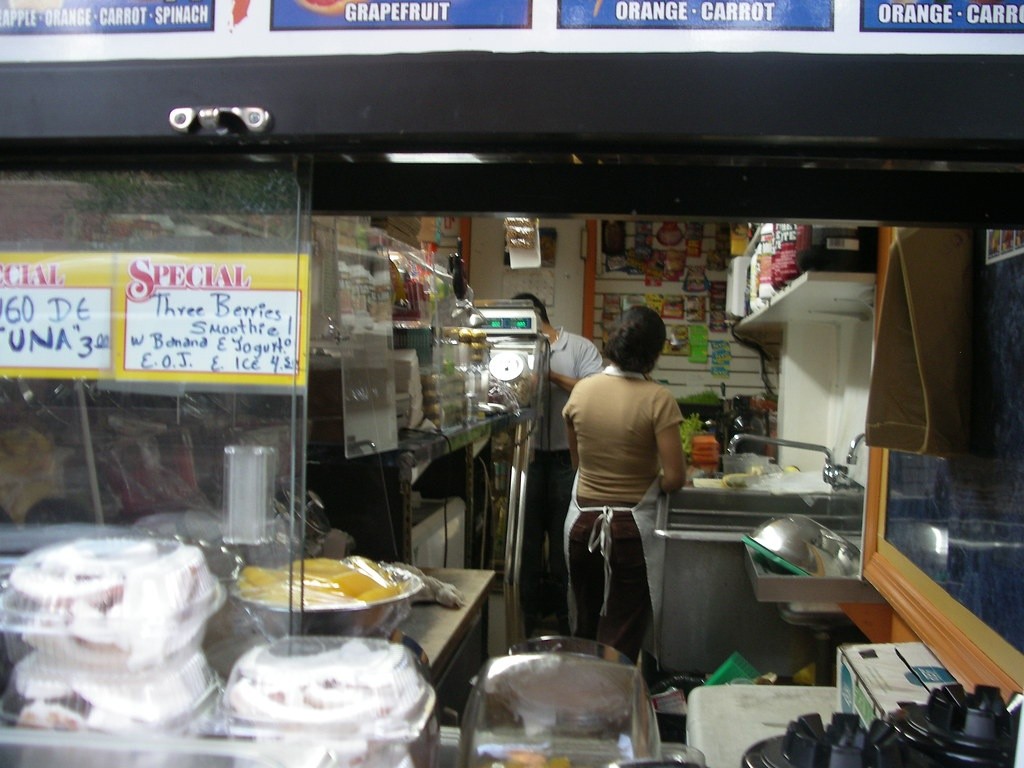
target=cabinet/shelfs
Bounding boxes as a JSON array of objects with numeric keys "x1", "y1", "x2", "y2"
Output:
[{"x1": 0, "y1": 209, "x2": 543, "y2": 597}]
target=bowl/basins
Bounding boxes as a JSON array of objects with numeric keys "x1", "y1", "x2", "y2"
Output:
[
  {"x1": 741, "y1": 514, "x2": 861, "y2": 576},
  {"x1": 509, "y1": 635, "x2": 638, "y2": 671},
  {"x1": 228, "y1": 563, "x2": 423, "y2": 644}
]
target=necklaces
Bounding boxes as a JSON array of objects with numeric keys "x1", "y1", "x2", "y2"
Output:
[{"x1": 555, "y1": 330, "x2": 559, "y2": 340}]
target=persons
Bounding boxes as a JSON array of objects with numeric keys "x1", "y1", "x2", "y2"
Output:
[
  {"x1": 561, "y1": 303, "x2": 685, "y2": 659},
  {"x1": 512, "y1": 293, "x2": 604, "y2": 634}
]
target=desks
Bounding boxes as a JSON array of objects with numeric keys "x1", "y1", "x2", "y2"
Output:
[{"x1": 388, "y1": 568, "x2": 496, "y2": 728}]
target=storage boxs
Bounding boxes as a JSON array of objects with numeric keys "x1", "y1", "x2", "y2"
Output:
[
  {"x1": 0, "y1": 538, "x2": 437, "y2": 768},
  {"x1": 838, "y1": 642, "x2": 960, "y2": 733}
]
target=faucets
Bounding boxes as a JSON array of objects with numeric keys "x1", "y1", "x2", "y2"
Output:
[
  {"x1": 726, "y1": 433, "x2": 836, "y2": 487},
  {"x1": 845, "y1": 432, "x2": 864, "y2": 465}
]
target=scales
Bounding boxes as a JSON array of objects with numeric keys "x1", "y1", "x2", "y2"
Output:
[{"x1": 472, "y1": 299, "x2": 543, "y2": 337}]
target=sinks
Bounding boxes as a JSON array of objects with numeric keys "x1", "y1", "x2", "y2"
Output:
[
  {"x1": 669, "y1": 488, "x2": 840, "y2": 516},
  {"x1": 666, "y1": 511, "x2": 854, "y2": 531}
]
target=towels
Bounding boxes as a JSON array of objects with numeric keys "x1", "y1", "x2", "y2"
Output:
[{"x1": 865, "y1": 228, "x2": 987, "y2": 459}]
